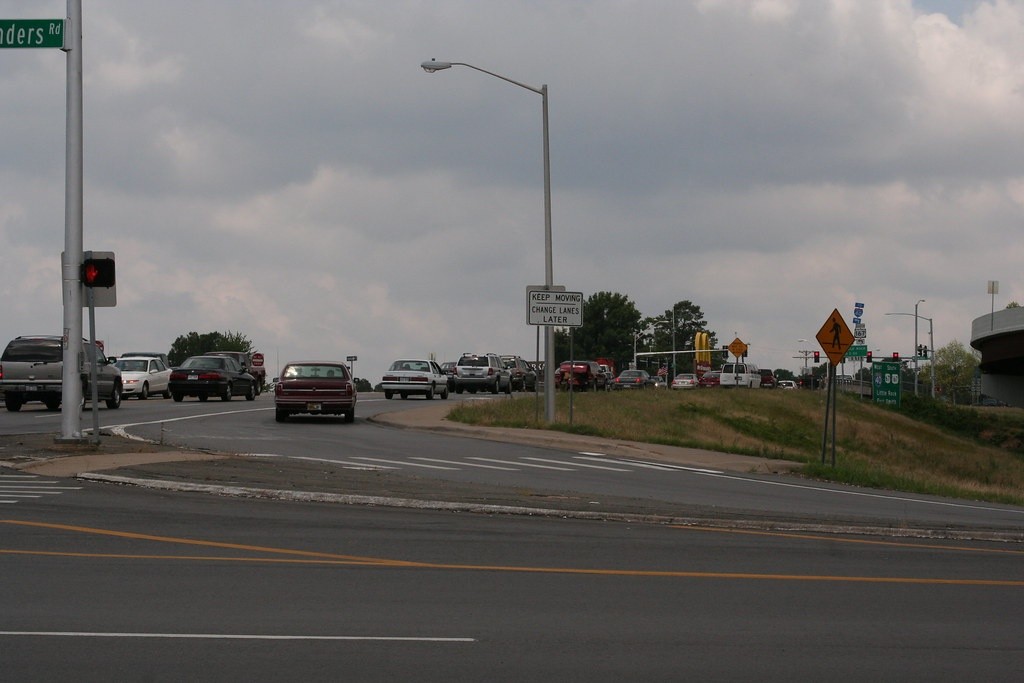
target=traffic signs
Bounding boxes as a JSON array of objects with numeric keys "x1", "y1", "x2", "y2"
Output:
[{"x1": 346, "y1": 356, "x2": 357, "y2": 362}]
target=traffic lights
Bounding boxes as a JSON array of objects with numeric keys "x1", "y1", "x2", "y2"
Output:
[
  {"x1": 893, "y1": 352, "x2": 899, "y2": 362},
  {"x1": 922, "y1": 345, "x2": 927, "y2": 358},
  {"x1": 814, "y1": 351, "x2": 819, "y2": 363},
  {"x1": 82, "y1": 259, "x2": 116, "y2": 288},
  {"x1": 866, "y1": 351, "x2": 873, "y2": 363},
  {"x1": 918, "y1": 344, "x2": 922, "y2": 357}
]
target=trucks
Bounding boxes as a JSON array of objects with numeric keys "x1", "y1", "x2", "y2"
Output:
[{"x1": 555, "y1": 356, "x2": 617, "y2": 393}]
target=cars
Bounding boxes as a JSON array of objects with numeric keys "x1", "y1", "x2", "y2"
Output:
[
  {"x1": 615, "y1": 370, "x2": 651, "y2": 391},
  {"x1": 110, "y1": 356, "x2": 175, "y2": 400},
  {"x1": 272, "y1": 360, "x2": 360, "y2": 424},
  {"x1": 439, "y1": 362, "x2": 458, "y2": 393},
  {"x1": 699, "y1": 371, "x2": 720, "y2": 388},
  {"x1": 381, "y1": 359, "x2": 449, "y2": 400},
  {"x1": 167, "y1": 355, "x2": 258, "y2": 402},
  {"x1": 672, "y1": 373, "x2": 699, "y2": 390},
  {"x1": 650, "y1": 376, "x2": 668, "y2": 391},
  {"x1": 778, "y1": 381, "x2": 799, "y2": 391}
]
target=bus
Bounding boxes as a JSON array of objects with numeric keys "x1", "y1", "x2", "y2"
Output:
[{"x1": 836, "y1": 374, "x2": 852, "y2": 386}]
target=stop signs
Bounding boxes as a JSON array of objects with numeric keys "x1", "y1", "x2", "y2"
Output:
[{"x1": 251, "y1": 353, "x2": 264, "y2": 367}]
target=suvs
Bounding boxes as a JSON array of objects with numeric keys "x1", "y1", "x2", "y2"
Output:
[
  {"x1": 453, "y1": 351, "x2": 512, "y2": 394},
  {"x1": 499, "y1": 354, "x2": 538, "y2": 393},
  {"x1": 0, "y1": 334, "x2": 124, "y2": 412},
  {"x1": 798, "y1": 374, "x2": 825, "y2": 389}
]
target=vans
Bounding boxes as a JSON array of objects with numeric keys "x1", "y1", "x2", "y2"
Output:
[
  {"x1": 758, "y1": 368, "x2": 779, "y2": 387},
  {"x1": 119, "y1": 352, "x2": 170, "y2": 368},
  {"x1": 720, "y1": 362, "x2": 761, "y2": 389},
  {"x1": 203, "y1": 351, "x2": 250, "y2": 373}
]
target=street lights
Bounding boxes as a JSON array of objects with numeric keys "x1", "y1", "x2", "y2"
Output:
[
  {"x1": 914, "y1": 299, "x2": 926, "y2": 398},
  {"x1": 420, "y1": 59, "x2": 556, "y2": 430},
  {"x1": 884, "y1": 312, "x2": 935, "y2": 399},
  {"x1": 633, "y1": 321, "x2": 668, "y2": 370}
]
